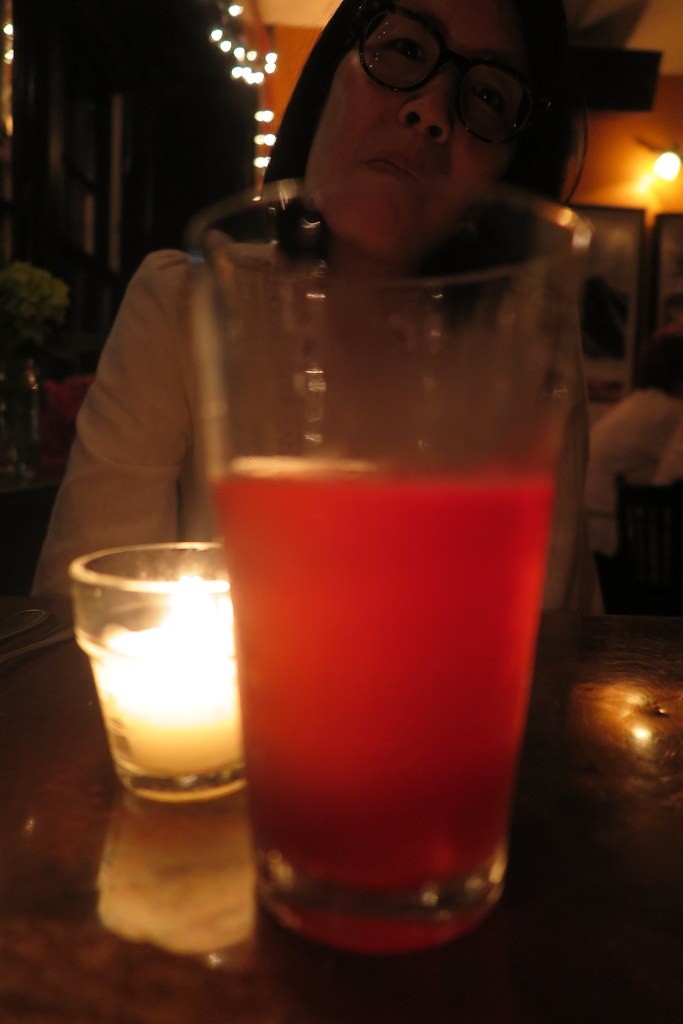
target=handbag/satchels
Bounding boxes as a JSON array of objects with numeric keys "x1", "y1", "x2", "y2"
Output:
[{"x1": 38, "y1": 374, "x2": 96, "y2": 474}]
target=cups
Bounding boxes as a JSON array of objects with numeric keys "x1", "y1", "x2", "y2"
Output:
[{"x1": 202, "y1": 186, "x2": 575, "y2": 943}]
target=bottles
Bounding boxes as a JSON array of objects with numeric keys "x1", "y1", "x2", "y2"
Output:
[{"x1": 11, "y1": 359, "x2": 41, "y2": 445}]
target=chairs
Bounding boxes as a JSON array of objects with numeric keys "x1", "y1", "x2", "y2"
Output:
[{"x1": 614, "y1": 472, "x2": 682, "y2": 619}]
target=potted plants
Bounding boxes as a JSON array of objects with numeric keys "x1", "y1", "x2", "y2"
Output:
[{"x1": 1, "y1": 260, "x2": 69, "y2": 466}]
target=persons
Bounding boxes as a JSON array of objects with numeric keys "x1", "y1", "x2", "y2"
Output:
[
  {"x1": 576, "y1": 325, "x2": 683, "y2": 603},
  {"x1": 29, "y1": 0, "x2": 606, "y2": 617}
]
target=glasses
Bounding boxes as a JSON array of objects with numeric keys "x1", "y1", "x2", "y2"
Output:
[{"x1": 342, "y1": 0, "x2": 553, "y2": 145}]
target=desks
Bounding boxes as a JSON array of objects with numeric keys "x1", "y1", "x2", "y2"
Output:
[{"x1": 0, "y1": 591, "x2": 682, "y2": 1024}]
